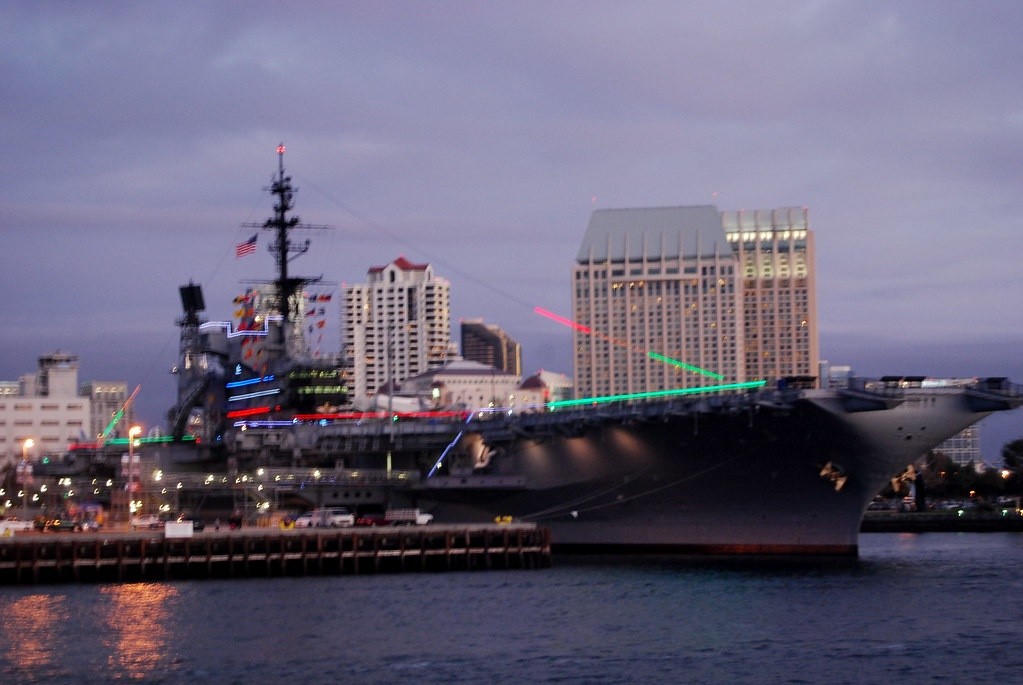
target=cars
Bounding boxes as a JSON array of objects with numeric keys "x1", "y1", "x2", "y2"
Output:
[
  {"x1": 128, "y1": 513, "x2": 161, "y2": 529},
  {"x1": 358, "y1": 513, "x2": 387, "y2": 529}
]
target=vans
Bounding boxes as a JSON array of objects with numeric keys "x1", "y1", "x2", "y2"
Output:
[{"x1": 311, "y1": 505, "x2": 357, "y2": 528}]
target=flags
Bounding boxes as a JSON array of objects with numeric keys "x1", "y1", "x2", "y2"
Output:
[
  {"x1": 235, "y1": 231, "x2": 259, "y2": 256},
  {"x1": 232, "y1": 288, "x2": 333, "y2": 378}
]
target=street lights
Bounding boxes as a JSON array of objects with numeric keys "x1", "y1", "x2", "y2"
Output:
[
  {"x1": 129, "y1": 425, "x2": 143, "y2": 515},
  {"x1": 22, "y1": 438, "x2": 35, "y2": 520}
]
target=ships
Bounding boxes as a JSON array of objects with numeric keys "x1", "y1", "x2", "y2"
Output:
[{"x1": 22, "y1": 131, "x2": 1023, "y2": 566}]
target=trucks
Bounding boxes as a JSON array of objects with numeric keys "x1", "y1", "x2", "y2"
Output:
[{"x1": 383, "y1": 507, "x2": 432, "y2": 526}]
